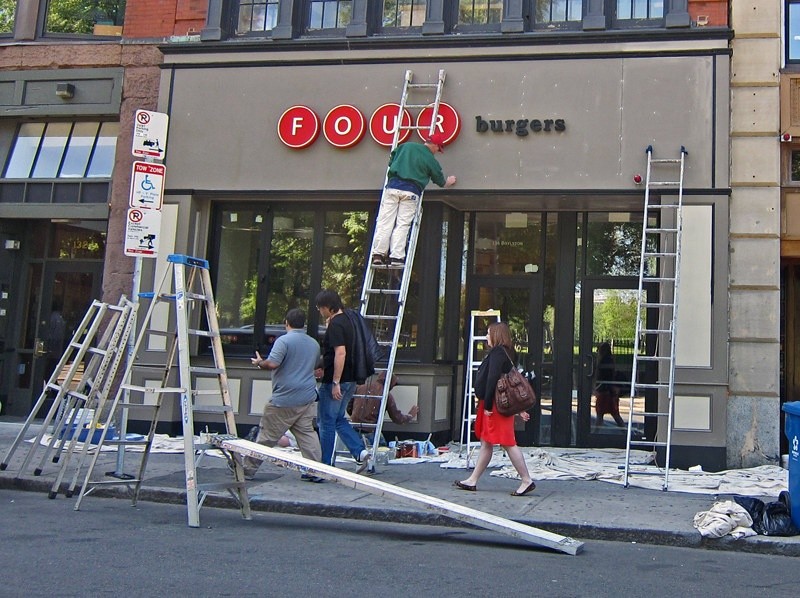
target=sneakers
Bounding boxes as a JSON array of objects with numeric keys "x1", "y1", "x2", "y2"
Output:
[{"x1": 355, "y1": 450, "x2": 370, "y2": 474}]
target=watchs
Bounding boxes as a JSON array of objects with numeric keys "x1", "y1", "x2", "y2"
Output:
[{"x1": 332, "y1": 382, "x2": 338, "y2": 386}]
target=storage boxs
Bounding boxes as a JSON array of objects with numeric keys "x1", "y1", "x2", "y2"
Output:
[{"x1": 60, "y1": 422, "x2": 116, "y2": 444}]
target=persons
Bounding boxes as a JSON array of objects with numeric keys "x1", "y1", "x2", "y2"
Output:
[
  {"x1": 227, "y1": 308, "x2": 321, "y2": 481},
  {"x1": 454, "y1": 322, "x2": 536, "y2": 496},
  {"x1": 347, "y1": 371, "x2": 420, "y2": 447},
  {"x1": 315, "y1": 288, "x2": 370, "y2": 474},
  {"x1": 596, "y1": 341, "x2": 626, "y2": 427},
  {"x1": 373, "y1": 134, "x2": 456, "y2": 265},
  {"x1": 43, "y1": 300, "x2": 64, "y2": 397}
]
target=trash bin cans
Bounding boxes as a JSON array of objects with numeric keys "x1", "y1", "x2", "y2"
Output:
[{"x1": 782, "y1": 402, "x2": 800, "y2": 531}]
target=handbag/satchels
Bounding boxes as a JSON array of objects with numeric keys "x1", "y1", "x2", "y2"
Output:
[{"x1": 493, "y1": 346, "x2": 538, "y2": 417}]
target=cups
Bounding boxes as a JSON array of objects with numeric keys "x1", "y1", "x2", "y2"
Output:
[
  {"x1": 782, "y1": 454, "x2": 789, "y2": 469},
  {"x1": 689, "y1": 465, "x2": 702, "y2": 471}
]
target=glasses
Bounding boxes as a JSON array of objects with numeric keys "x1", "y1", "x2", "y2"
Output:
[{"x1": 315, "y1": 304, "x2": 320, "y2": 311}]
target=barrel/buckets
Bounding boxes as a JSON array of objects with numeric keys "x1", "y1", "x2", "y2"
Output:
[
  {"x1": 65, "y1": 407, "x2": 95, "y2": 425},
  {"x1": 376, "y1": 440, "x2": 429, "y2": 467}
]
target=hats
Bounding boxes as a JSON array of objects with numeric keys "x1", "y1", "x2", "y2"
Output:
[{"x1": 426, "y1": 134, "x2": 444, "y2": 154}]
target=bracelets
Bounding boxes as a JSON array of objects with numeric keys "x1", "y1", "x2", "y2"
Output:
[{"x1": 258, "y1": 364, "x2": 261, "y2": 369}]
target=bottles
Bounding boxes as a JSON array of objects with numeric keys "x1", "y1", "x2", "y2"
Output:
[{"x1": 438, "y1": 447, "x2": 449, "y2": 455}]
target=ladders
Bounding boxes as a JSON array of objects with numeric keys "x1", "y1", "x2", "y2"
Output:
[
  {"x1": 1, "y1": 292, "x2": 139, "y2": 500},
  {"x1": 330, "y1": 69, "x2": 447, "y2": 472},
  {"x1": 74, "y1": 255, "x2": 252, "y2": 527},
  {"x1": 623, "y1": 145, "x2": 687, "y2": 491},
  {"x1": 459, "y1": 310, "x2": 507, "y2": 470}
]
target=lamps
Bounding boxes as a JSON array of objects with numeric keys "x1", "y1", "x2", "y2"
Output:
[{"x1": 56, "y1": 83, "x2": 74, "y2": 98}]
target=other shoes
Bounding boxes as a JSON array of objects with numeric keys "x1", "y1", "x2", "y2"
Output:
[
  {"x1": 227, "y1": 462, "x2": 256, "y2": 480},
  {"x1": 390, "y1": 258, "x2": 404, "y2": 266},
  {"x1": 372, "y1": 254, "x2": 386, "y2": 265},
  {"x1": 453, "y1": 479, "x2": 477, "y2": 492},
  {"x1": 300, "y1": 473, "x2": 325, "y2": 483},
  {"x1": 509, "y1": 481, "x2": 537, "y2": 496}
]
